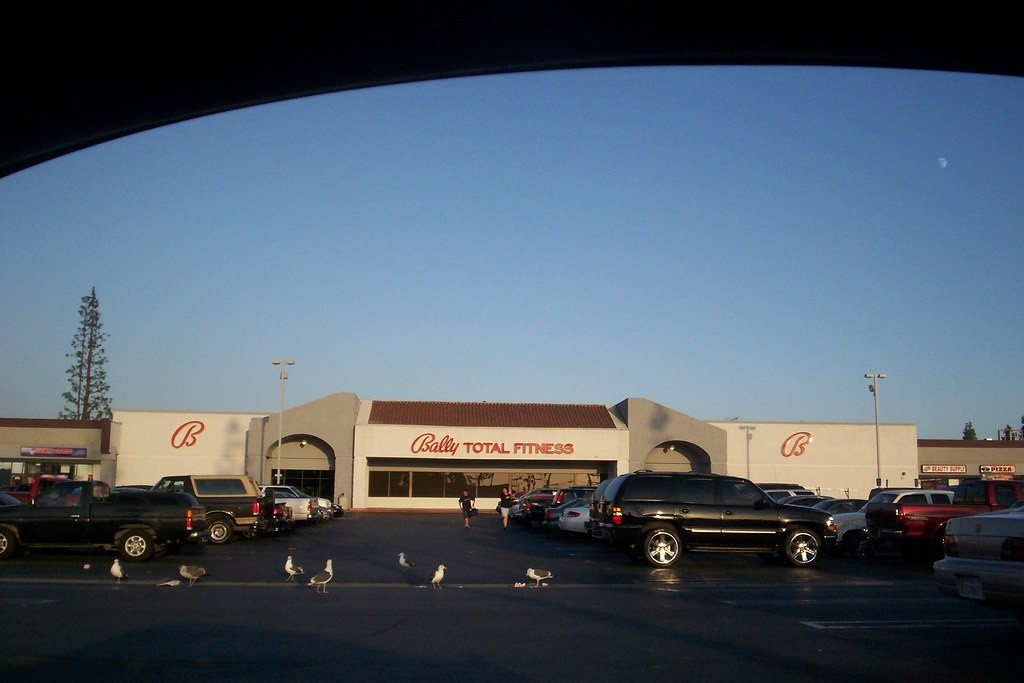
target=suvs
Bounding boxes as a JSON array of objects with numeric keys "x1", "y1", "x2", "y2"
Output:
[
  {"x1": 148, "y1": 474, "x2": 265, "y2": 544},
  {"x1": 597, "y1": 468, "x2": 836, "y2": 569}
]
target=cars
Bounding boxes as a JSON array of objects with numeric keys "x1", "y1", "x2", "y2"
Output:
[
  {"x1": 498, "y1": 487, "x2": 593, "y2": 544},
  {"x1": 933, "y1": 500, "x2": 1024, "y2": 625},
  {"x1": 757, "y1": 483, "x2": 957, "y2": 558},
  {"x1": 259, "y1": 484, "x2": 344, "y2": 534}
]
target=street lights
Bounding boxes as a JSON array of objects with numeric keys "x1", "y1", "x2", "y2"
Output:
[
  {"x1": 864, "y1": 374, "x2": 886, "y2": 489},
  {"x1": 273, "y1": 361, "x2": 295, "y2": 483}
]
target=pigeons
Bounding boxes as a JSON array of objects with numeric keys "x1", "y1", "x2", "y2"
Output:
[
  {"x1": 525, "y1": 568, "x2": 555, "y2": 587},
  {"x1": 304, "y1": 558, "x2": 334, "y2": 593},
  {"x1": 397, "y1": 552, "x2": 416, "y2": 569},
  {"x1": 110, "y1": 559, "x2": 128, "y2": 584},
  {"x1": 428, "y1": 564, "x2": 448, "y2": 589},
  {"x1": 284, "y1": 555, "x2": 304, "y2": 582},
  {"x1": 178, "y1": 564, "x2": 211, "y2": 587}
]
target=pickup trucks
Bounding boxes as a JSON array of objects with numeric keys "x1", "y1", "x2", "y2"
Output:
[
  {"x1": 0, "y1": 480, "x2": 206, "y2": 563},
  {"x1": 867, "y1": 480, "x2": 1024, "y2": 562},
  {"x1": 4, "y1": 475, "x2": 81, "y2": 506}
]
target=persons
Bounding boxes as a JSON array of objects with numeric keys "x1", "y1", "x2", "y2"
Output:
[
  {"x1": 458, "y1": 490, "x2": 475, "y2": 527},
  {"x1": 500, "y1": 487, "x2": 515, "y2": 528}
]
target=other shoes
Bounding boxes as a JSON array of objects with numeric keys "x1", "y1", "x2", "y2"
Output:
[{"x1": 465, "y1": 525, "x2": 469, "y2": 528}]
